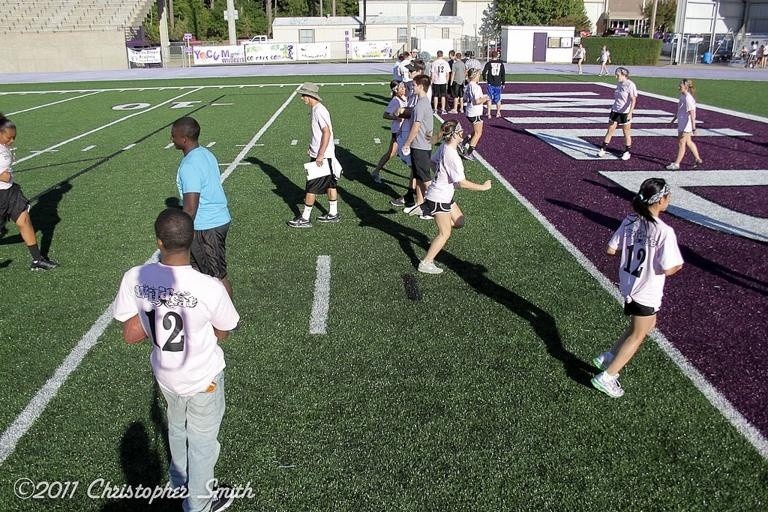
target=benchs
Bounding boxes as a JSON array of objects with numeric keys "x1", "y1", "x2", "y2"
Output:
[{"x1": 0, "y1": 0, "x2": 157, "y2": 42}]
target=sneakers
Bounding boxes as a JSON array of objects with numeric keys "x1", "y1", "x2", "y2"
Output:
[
  {"x1": 463, "y1": 152, "x2": 475, "y2": 160},
  {"x1": 370, "y1": 172, "x2": 385, "y2": 183},
  {"x1": 666, "y1": 163, "x2": 679, "y2": 170},
  {"x1": 597, "y1": 148, "x2": 606, "y2": 157},
  {"x1": 457, "y1": 142, "x2": 465, "y2": 153},
  {"x1": 288, "y1": 215, "x2": 313, "y2": 228},
  {"x1": 432, "y1": 108, "x2": 464, "y2": 117},
  {"x1": 315, "y1": 212, "x2": 339, "y2": 223},
  {"x1": 418, "y1": 260, "x2": 443, "y2": 274},
  {"x1": 485, "y1": 112, "x2": 501, "y2": 119},
  {"x1": 689, "y1": 159, "x2": 702, "y2": 168},
  {"x1": 391, "y1": 196, "x2": 435, "y2": 219},
  {"x1": 30, "y1": 258, "x2": 59, "y2": 272},
  {"x1": 590, "y1": 351, "x2": 624, "y2": 397},
  {"x1": 211, "y1": 483, "x2": 235, "y2": 512},
  {"x1": 622, "y1": 152, "x2": 631, "y2": 160}
]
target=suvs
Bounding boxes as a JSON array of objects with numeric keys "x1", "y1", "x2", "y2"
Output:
[{"x1": 606, "y1": 28, "x2": 631, "y2": 37}]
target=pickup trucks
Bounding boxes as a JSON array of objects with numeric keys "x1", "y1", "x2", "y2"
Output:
[{"x1": 241, "y1": 34, "x2": 273, "y2": 45}]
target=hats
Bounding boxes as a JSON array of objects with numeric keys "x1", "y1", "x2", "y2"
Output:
[
  {"x1": 410, "y1": 49, "x2": 419, "y2": 53},
  {"x1": 468, "y1": 68, "x2": 480, "y2": 79},
  {"x1": 297, "y1": 82, "x2": 323, "y2": 101}
]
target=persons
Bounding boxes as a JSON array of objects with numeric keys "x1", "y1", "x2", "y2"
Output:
[
  {"x1": 372, "y1": 80, "x2": 407, "y2": 183},
  {"x1": 459, "y1": 68, "x2": 489, "y2": 159},
  {"x1": 112, "y1": 208, "x2": 239, "y2": 512},
  {"x1": 664, "y1": 77, "x2": 704, "y2": 170},
  {"x1": 409, "y1": 120, "x2": 493, "y2": 276},
  {"x1": 574, "y1": 43, "x2": 587, "y2": 75},
  {"x1": 286, "y1": 81, "x2": 342, "y2": 229},
  {"x1": 0, "y1": 112, "x2": 58, "y2": 272},
  {"x1": 738, "y1": 40, "x2": 767, "y2": 69},
  {"x1": 169, "y1": 116, "x2": 233, "y2": 340},
  {"x1": 390, "y1": 60, "x2": 432, "y2": 207},
  {"x1": 401, "y1": 76, "x2": 433, "y2": 220},
  {"x1": 596, "y1": 45, "x2": 612, "y2": 77},
  {"x1": 591, "y1": 178, "x2": 685, "y2": 399},
  {"x1": 391, "y1": 49, "x2": 506, "y2": 118},
  {"x1": 596, "y1": 67, "x2": 637, "y2": 161}
]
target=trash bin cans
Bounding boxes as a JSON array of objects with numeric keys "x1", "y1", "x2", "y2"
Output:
[{"x1": 701, "y1": 51, "x2": 712, "y2": 64}]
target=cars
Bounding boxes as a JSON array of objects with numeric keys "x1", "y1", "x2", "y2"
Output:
[
  {"x1": 483, "y1": 43, "x2": 502, "y2": 60},
  {"x1": 653, "y1": 32, "x2": 731, "y2": 66},
  {"x1": 573, "y1": 24, "x2": 593, "y2": 46}
]
